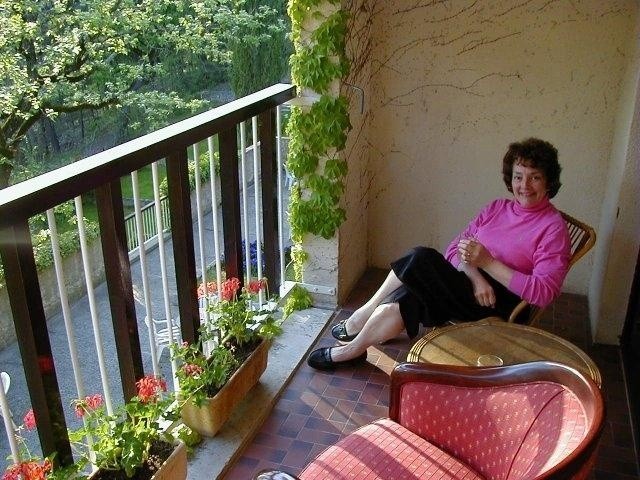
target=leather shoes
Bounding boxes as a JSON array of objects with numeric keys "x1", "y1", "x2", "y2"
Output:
[
  {"x1": 331, "y1": 318, "x2": 363, "y2": 341},
  {"x1": 308, "y1": 343, "x2": 366, "y2": 371}
]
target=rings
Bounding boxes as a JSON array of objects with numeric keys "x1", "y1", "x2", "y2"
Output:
[{"x1": 467, "y1": 253, "x2": 470, "y2": 257}]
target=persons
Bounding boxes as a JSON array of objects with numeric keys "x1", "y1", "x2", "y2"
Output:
[{"x1": 303, "y1": 136, "x2": 572, "y2": 372}]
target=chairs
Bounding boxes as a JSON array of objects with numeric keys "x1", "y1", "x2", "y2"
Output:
[
  {"x1": 0, "y1": 371, "x2": 18, "y2": 431},
  {"x1": 436, "y1": 211, "x2": 596, "y2": 326},
  {"x1": 144, "y1": 315, "x2": 182, "y2": 365},
  {"x1": 253, "y1": 361, "x2": 607, "y2": 480}
]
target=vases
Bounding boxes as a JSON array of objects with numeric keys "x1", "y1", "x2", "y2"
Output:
[
  {"x1": 85, "y1": 438, "x2": 187, "y2": 480},
  {"x1": 181, "y1": 335, "x2": 268, "y2": 437}
]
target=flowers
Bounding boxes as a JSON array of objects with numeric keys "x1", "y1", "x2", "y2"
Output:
[
  {"x1": 171, "y1": 277, "x2": 284, "y2": 407},
  {"x1": 0, "y1": 375, "x2": 173, "y2": 480}
]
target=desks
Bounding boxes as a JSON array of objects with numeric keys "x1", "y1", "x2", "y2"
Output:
[{"x1": 406, "y1": 322, "x2": 602, "y2": 388}]
